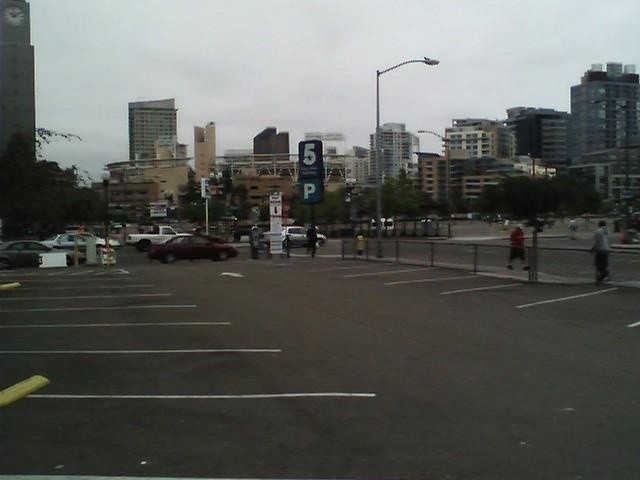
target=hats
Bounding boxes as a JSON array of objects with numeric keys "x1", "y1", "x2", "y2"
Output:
[{"x1": 516, "y1": 223, "x2": 525, "y2": 229}]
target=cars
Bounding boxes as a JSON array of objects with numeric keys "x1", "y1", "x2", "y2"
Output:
[
  {"x1": 262, "y1": 225, "x2": 328, "y2": 248},
  {"x1": 51, "y1": 230, "x2": 120, "y2": 252},
  {"x1": 1, "y1": 239, "x2": 87, "y2": 269},
  {"x1": 148, "y1": 234, "x2": 238, "y2": 264}
]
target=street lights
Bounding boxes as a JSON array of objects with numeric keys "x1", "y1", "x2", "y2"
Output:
[
  {"x1": 589, "y1": 96, "x2": 632, "y2": 228},
  {"x1": 100, "y1": 166, "x2": 112, "y2": 268},
  {"x1": 374, "y1": 57, "x2": 439, "y2": 258},
  {"x1": 417, "y1": 130, "x2": 450, "y2": 201}
]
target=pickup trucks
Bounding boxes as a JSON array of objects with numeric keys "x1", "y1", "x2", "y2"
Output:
[{"x1": 125, "y1": 225, "x2": 194, "y2": 252}]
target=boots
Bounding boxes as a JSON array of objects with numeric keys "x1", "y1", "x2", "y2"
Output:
[
  {"x1": 507, "y1": 265, "x2": 513, "y2": 269},
  {"x1": 523, "y1": 266, "x2": 530, "y2": 271}
]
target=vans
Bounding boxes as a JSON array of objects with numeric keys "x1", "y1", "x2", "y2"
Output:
[{"x1": 371, "y1": 218, "x2": 394, "y2": 233}]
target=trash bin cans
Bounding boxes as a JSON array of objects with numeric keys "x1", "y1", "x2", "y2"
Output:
[{"x1": 420, "y1": 219, "x2": 432, "y2": 235}]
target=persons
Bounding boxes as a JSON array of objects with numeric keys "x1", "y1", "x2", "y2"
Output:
[
  {"x1": 568, "y1": 217, "x2": 577, "y2": 241},
  {"x1": 304, "y1": 224, "x2": 318, "y2": 259},
  {"x1": 505, "y1": 223, "x2": 529, "y2": 272},
  {"x1": 588, "y1": 219, "x2": 612, "y2": 284}
]
